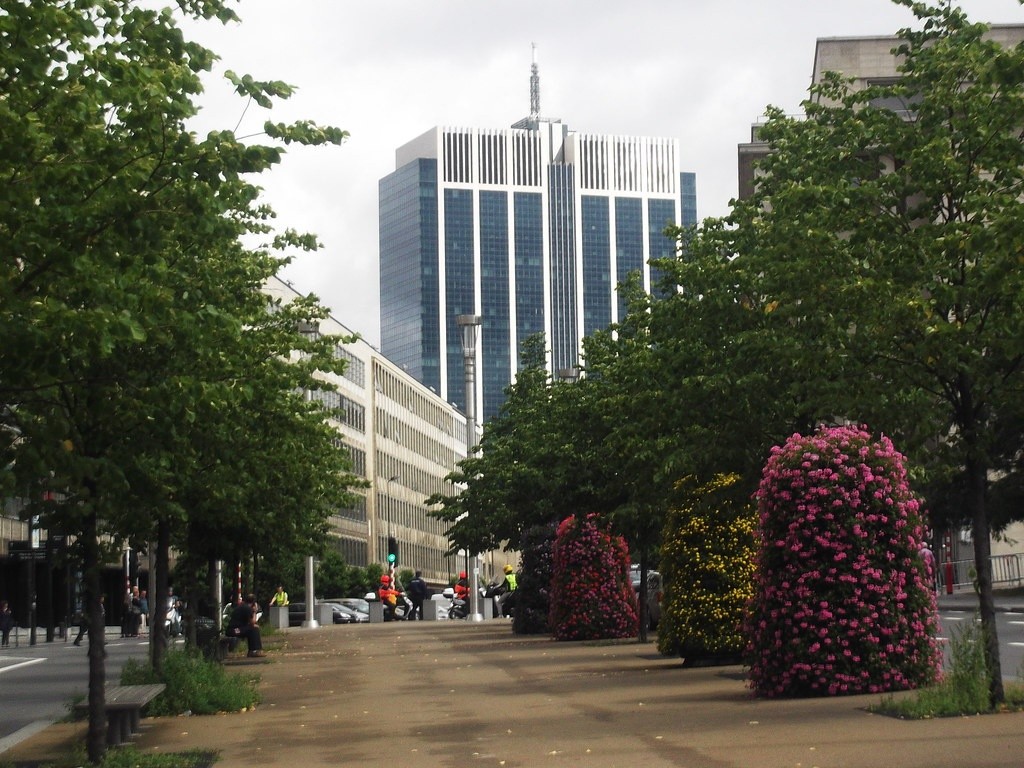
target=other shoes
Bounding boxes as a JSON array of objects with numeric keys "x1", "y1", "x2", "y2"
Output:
[
  {"x1": 498, "y1": 615, "x2": 504, "y2": 618},
  {"x1": 2, "y1": 645, "x2": 10, "y2": 648},
  {"x1": 247, "y1": 649, "x2": 267, "y2": 657},
  {"x1": 122, "y1": 632, "x2": 138, "y2": 638},
  {"x1": 74, "y1": 640, "x2": 81, "y2": 646}
]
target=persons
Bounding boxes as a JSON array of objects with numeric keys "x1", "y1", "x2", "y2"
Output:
[
  {"x1": 408, "y1": 571, "x2": 429, "y2": 621},
  {"x1": 167, "y1": 587, "x2": 197, "y2": 647},
  {"x1": 100, "y1": 596, "x2": 108, "y2": 645},
  {"x1": 0, "y1": 600, "x2": 14, "y2": 647},
  {"x1": 74, "y1": 598, "x2": 88, "y2": 645},
  {"x1": 455, "y1": 571, "x2": 470, "y2": 613},
  {"x1": 496, "y1": 565, "x2": 517, "y2": 619},
  {"x1": 270, "y1": 586, "x2": 289, "y2": 607},
  {"x1": 120, "y1": 586, "x2": 147, "y2": 638},
  {"x1": 378, "y1": 575, "x2": 402, "y2": 621},
  {"x1": 226, "y1": 594, "x2": 267, "y2": 658}
]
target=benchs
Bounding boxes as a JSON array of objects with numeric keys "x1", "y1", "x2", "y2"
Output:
[
  {"x1": 222, "y1": 636, "x2": 238, "y2": 655},
  {"x1": 76, "y1": 684, "x2": 168, "y2": 745}
]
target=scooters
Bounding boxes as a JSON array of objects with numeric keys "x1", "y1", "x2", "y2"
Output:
[
  {"x1": 442, "y1": 588, "x2": 471, "y2": 621},
  {"x1": 164, "y1": 602, "x2": 183, "y2": 638},
  {"x1": 486, "y1": 575, "x2": 518, "y2": 619}
]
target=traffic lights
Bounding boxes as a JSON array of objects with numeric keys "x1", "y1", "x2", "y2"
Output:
[
  {"x1": 133, "y1": 548, "x2": 142, "y2": 578},
  {"x1": 389, "y1": 539, "x2": 395, "y2": 562}
]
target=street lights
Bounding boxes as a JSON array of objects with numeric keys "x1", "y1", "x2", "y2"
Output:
[
  {"x1": 454, "y1": 316, "x2": 483, "y2": 623},
  {"x1": 387, "y1": 475, "x2": 399, "y2": 537}
]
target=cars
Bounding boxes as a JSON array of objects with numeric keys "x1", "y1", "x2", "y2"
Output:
[
  {"x1": 185, "y1": 600, "x2": 262, "y2": 643},
  {"x1": 288, "y1": 593, "x2": 420, "y2": 626},
  {"x1": 627, "y1": 571, "x2": 663, "y2": 630},
  {"x1": 430, "y1": 594, "x2": 456, "y2": 620}
]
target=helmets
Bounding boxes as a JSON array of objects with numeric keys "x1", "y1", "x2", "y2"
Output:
[
  {"x1": 381, "y1": 575, "x2": 389, "y2": 582},
  {"x1": 503, "y1": 565, "x2": 513, "y2": 574},
  {"x1": 460, "y1": 572, "x2": 466, "y2": 578}
]
